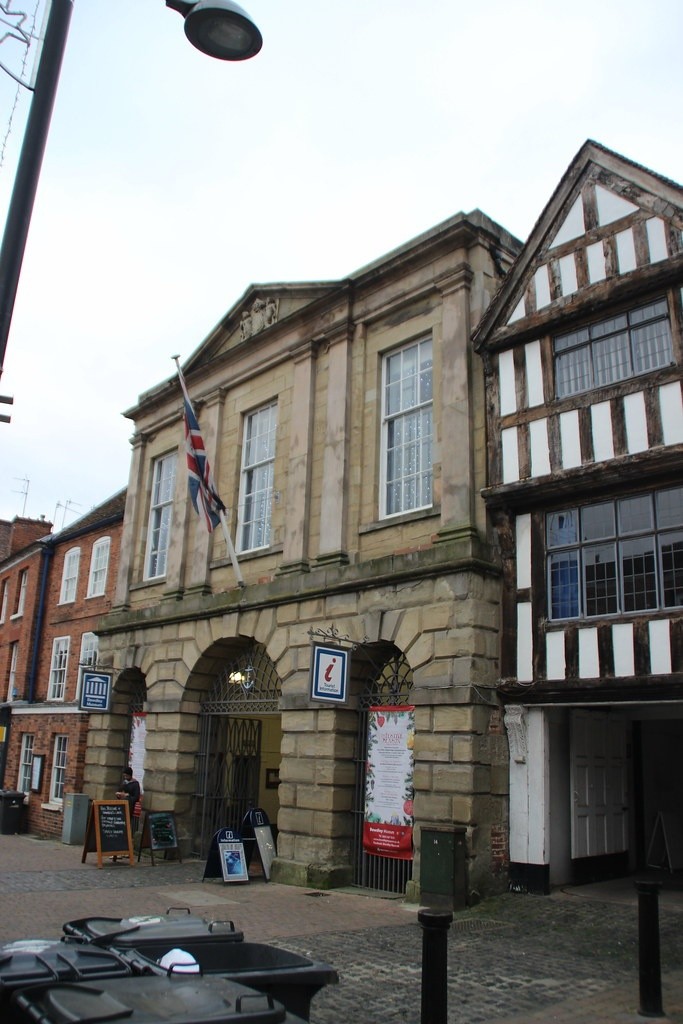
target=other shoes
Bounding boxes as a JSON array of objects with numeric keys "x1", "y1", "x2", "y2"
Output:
[
  {"x1": 122, "y1": 855, "x2": 130, "y2": 858},
  {"x1": 109, "y1": 854, "x2": 123, "y2": 858}
]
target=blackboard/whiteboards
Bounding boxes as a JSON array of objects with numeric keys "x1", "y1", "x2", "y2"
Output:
[
  {"x1": 646, "y1": 809, "x2": 683, "y2": 869},
  {"x1": 84, "y1": 800, "x2": 134, "y2": 856}
]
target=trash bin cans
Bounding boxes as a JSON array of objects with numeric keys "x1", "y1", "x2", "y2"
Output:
[
  {"x1": 108, "y1": 939, "x2": 339, "y2": 1023},
  {"x1": 63, "y1": 906, "x2": 244, "y2": 951},
  {"x1": 0, "y1": 789, "x2": 27, "y2": 835},
  {"x1": 9, "y1": 962, "x2": 310, "y2": 1024},
  {"x1": 0, "y1": 938, "x2": 132, "y2": 1024}
]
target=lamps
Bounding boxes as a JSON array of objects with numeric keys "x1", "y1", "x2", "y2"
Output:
[{"x1": 229, "y1": 657, "x2": 244, "y2": 685}]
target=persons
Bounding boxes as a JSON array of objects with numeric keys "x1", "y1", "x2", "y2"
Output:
[
  {"x1": 239, "y1": 296, "x2": 277, "y2": 342},
  {"x1": 114, "y1": 767, "x2": 140, "y2": 821}
]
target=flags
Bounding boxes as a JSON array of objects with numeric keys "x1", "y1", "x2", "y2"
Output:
[{"x1": 180, "y1": 373, "x2": 229, "y2": 533}]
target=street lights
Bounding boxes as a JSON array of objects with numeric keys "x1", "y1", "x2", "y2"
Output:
[{"x1": 2, "y1": 1, "x2": 265, "y2": 398}]
